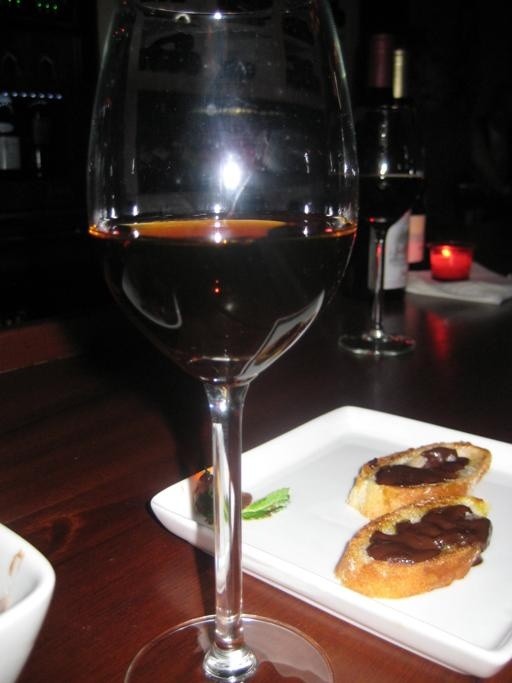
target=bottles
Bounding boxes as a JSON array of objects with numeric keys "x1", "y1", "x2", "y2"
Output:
[{"x1": 341, "y1": 48, "x2": 420, "y2": 359}]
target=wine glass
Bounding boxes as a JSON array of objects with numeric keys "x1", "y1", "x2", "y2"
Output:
[{"x1": 90, "y1": 1, "x2": 362, "y2": 682}]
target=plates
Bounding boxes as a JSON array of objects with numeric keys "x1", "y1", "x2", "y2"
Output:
[{"x1": 151, "y1": 403, "x2": 512, "y2": 681}]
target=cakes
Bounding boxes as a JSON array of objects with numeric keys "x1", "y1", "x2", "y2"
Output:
[
  {"x1": 348, "y1": 442, "x2": 492, "y2": 520},
  {"x1": 335, "y1": 496, "x2": 493, "y2": 600}
]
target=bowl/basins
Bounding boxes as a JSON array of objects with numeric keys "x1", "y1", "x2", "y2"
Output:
[{"x1": 0, "y1": 517, "x2": 57, "y2": 682}]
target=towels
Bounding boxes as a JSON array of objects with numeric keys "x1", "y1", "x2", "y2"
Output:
[{"x1": 404, "y1": 260, "x2": 512, "y2": 305}]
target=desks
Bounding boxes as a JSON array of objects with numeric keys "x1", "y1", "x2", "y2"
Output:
[{"x1": 0, "y1": 280, "x2": 512, "y2": 682}]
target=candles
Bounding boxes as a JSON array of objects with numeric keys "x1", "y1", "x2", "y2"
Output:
[{"x1": 429, "y1": 244, "x2": 473, "y2": 279}]
